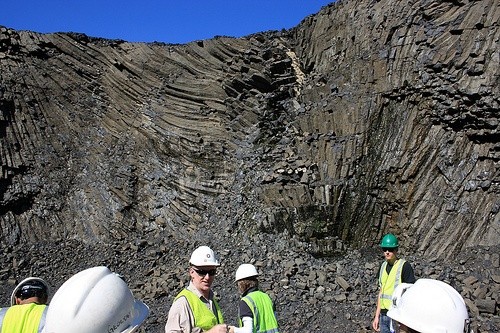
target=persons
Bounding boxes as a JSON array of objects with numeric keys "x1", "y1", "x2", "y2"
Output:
[
  {"x1": 164, "y1": 246, "x2": 228, "y2": 333},
  {"x1": 47, "y1": 266, "x2": 150, "y2": 333},
  {"x1": 228, "y1": 263, "x2": 280, "y2": 333},
  {"x1": 373, "y1": 233, "x2": 416, "y2": 333},
  {"x1": 0, "y1": 277, "x2": 49, "y2": 333},
  {"x1": 386, "y1": 278, "x2": 469, "y2": 333}
]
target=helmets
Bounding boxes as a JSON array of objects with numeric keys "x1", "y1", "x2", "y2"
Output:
[
  {"x1": 41, "y1": 266, "x2": 149, "y2": 333},
  {"x1": 189, "y1": 245, "x2": 220, "y2": 266},
  {"x1": 386, "y1": 279, "x2": 474, "y2": 333},
  {"x1": 11, "y1": 277, "x2": 48, "y2": 305},
  {"x1": 232, "y1": 264, "x2": 261, "y2": 284},
  {"x1": 379, "y1": 233, "x2": 400, "y2": 247}
]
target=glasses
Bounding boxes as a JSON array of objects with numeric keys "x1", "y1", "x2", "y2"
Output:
[
  {"x1": 381, "y1": 247, "x2": 394, "y2": 252},
  {"x1": 191, "y1": 267, "x2": 216, "y2": 276}
]
